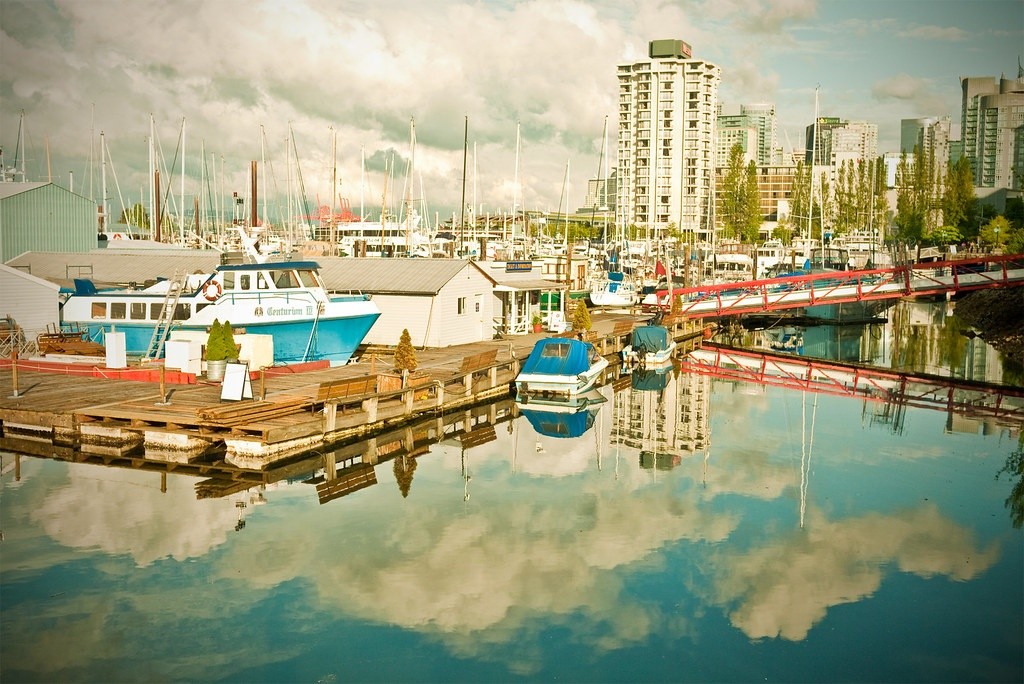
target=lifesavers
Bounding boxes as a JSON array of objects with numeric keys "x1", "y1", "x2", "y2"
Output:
[
  {"x1": 202, "y1": 281, "x2": 222, "y2": 300},
  {"x1": 114, "y1": 234, "x2": 121, "y2": 240}
]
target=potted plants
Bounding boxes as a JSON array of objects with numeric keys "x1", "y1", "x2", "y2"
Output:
[
  {"x1": 530, "y1": 311, "x2": 543, "y2": 333},
  {"x1": 222, "y1": 320, "x2": 239, "y2": 364},
  {"x1": 206, "y1": 318, "x2": 229, "y2": 381},
  {"x1": 571, "y1": 299, "x2": 598, "y2": 342},
  {"x1": 377, "y1": 329, "x2": 431, "y2": 401}
]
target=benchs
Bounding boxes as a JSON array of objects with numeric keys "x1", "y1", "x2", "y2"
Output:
[
  {"x1": 452, "y1": 348, "x2": 499, "y2": 387},
  {"x1": 661, "y1": 314, "x2": 676, "y2": 326},
  {"x1": 546, "y1": 331, "x2": 579, "y2": 339},
  {"x1": 306, "y1": 374, "x2": 378, "y2": 416},
  {"x1": 595, "y1": 307, "x2": 646, "y2": 315},
  {"x1": 493, "y1": 324, "x2": 512, "y2": 339},
  {"x1": 602, "y1": 320, "x2": 635, "y2": 338}
]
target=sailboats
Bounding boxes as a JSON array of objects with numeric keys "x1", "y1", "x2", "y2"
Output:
[{"x1": 54, "y1": 115, "x2": 815, "y2": 375}]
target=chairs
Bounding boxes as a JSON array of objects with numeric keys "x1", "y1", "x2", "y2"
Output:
[{"x1": 551, "y1": 312, "x2": 565, "y2": 333}]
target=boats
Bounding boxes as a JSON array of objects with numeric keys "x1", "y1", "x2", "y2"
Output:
[
  {"x1": 621, "y1": 357, "x2": 678, "y2": 391},
  {"x1": 514, "y1": 385, "x2": 609, "y2": 438},
  {"x1": 513, "y1": 337, "x2": 609, "y2": 395},
  {"x1": 622, "y1": 326, "x2": 678, "y2": 364}
]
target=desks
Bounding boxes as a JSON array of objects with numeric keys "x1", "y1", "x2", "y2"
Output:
[{"x1": 493, "y1": 316, "x2": 505, "y2": 319}]
